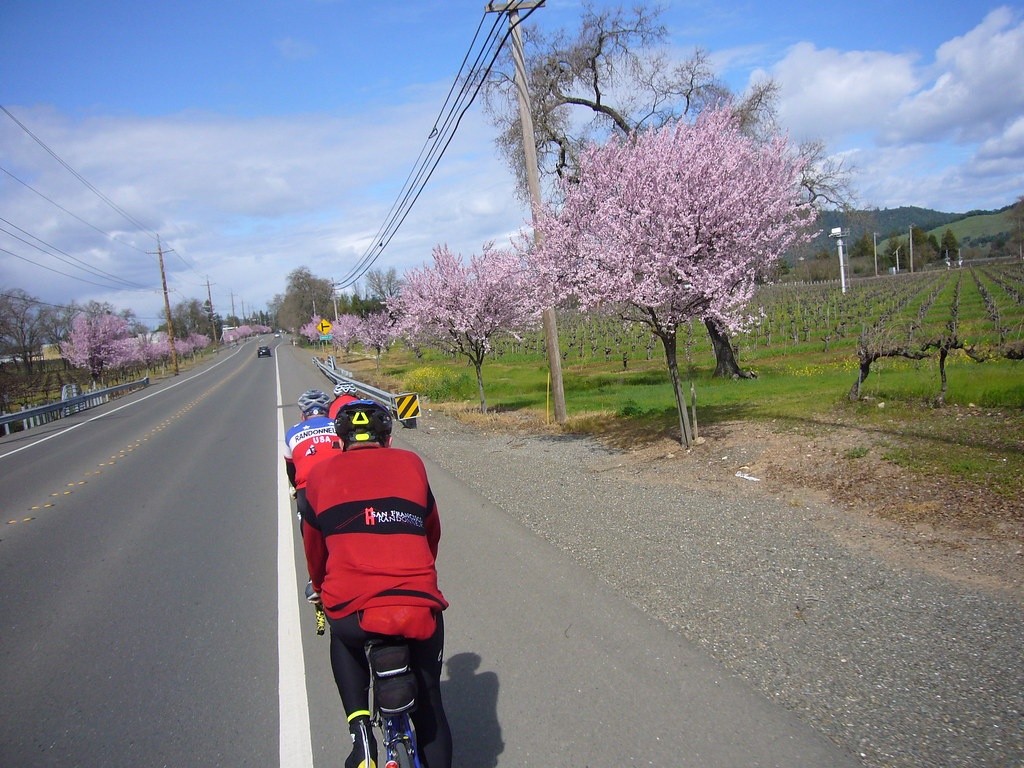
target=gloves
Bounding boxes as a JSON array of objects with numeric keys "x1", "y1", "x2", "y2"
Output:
[
  {"x1": 305, "y1": 581, "x2": 322, "y2": 605},
  {"x1": 289, "y1": 487, "x2": 297, "y2": 499}
]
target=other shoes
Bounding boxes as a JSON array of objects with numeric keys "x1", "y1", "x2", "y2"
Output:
[{"x1": 344, "y1": 724, "x2": 378, "y2": 768}]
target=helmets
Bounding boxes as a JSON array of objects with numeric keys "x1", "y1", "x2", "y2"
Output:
[
  {"x1": 333, "y1": 381, "x2": 358, "y2": 397},
  {"x1": 334, "y1": 400, "x2": 392, "y2": 443},
  {"x1": 298, "y1": 390, "x2": 331, "y2": 412}
]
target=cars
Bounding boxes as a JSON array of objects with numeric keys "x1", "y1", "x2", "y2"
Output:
[{"x1": 256, "y1": 346, "x2": 271, "y2": 358}]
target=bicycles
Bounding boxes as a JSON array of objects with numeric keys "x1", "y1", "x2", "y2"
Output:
[{"x1": 315, "y1": 597, "x2": 430, "y2": 768}]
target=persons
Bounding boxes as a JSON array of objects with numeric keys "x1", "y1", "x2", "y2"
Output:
[
  {"x1": 298, "y1": 401, "x2": 452, "y2": 768},
  {"x1": 284, "y1": 389, "x2": 343, "y2": 539},
  {"x1": 328, "y1": 381, "x2": 360, "y2": 420}
]
